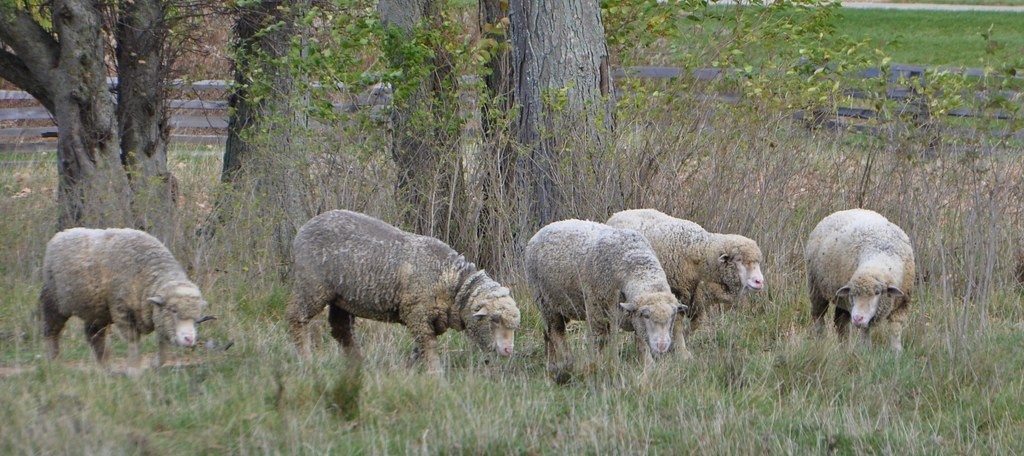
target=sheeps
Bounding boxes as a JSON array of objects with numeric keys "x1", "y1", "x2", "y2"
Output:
[{"x1": 41, "y1": 209, "x2": 916, "y2": 372}]
